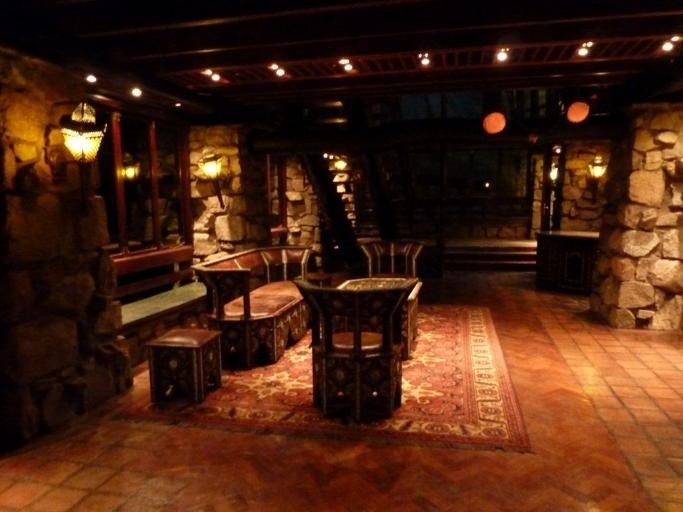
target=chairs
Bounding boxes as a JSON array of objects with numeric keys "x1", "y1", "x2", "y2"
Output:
[{"x1": 293, "y1": 225, "x2": 425, "y2": 420}]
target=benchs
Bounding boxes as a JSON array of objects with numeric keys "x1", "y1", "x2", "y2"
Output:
[
  {"x1": 113, "y1": 246, "x2": 207, "y2": 376},
  {"x1": 192, "y1": 246, "x2": 311, "y2": 368}
]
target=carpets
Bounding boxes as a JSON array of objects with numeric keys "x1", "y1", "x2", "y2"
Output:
[{"x1": 113, "y1": 303, "x2": 536, "y2": 452}]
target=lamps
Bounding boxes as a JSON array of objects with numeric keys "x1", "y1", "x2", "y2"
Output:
[
  {"x1": 197, "y1": 151, "x2": 224, "y2": 209},
  {"x1": 50, "y1": 98, "x2": 108, "y2": 166},
  {"x1": 586, "y1": 150, "x2": 609, "y2": 204}
]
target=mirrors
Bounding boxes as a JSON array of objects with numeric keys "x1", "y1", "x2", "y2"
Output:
[{"x1": 90, "y1": 94, "x2": 193, "y2": 251}]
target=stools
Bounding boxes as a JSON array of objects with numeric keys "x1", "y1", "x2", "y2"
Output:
[{"x1": 144, "y1": 328, "x2": 225, "y2": 403}]
